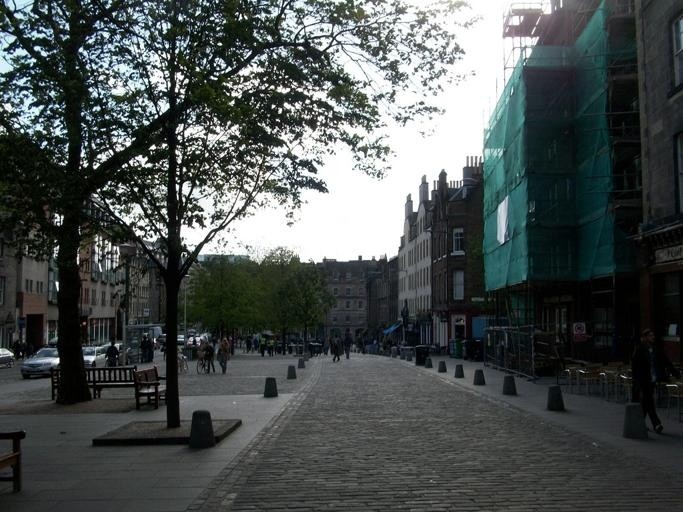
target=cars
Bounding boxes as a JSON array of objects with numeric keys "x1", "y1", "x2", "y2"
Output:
[
  {"x1": 156, "y1": 326, "x2": 211, "y2": 348},
  {"x1": 19, "y1": 343, "x2": 130, "y2": 378},
  {"x1": 0, "y1": 347, "x2": 14, "y2": 368}
]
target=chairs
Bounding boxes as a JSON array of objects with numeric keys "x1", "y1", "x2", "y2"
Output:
[{"x1": 558, "y1": 356, "x2": 682, "y2": 425}]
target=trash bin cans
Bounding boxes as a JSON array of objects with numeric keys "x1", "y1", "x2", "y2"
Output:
[
  {"x1": 448, "y1": 338, "x2": 482, "y2": 362},
  {"x1": 415, "y1": 345, "x2": 429, "y2": 366}
]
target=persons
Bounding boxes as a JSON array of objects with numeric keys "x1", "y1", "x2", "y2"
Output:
[
  {"x1": 632, "y1": 329, "x2": 680, "y2": 432},
  {"x1": 13, "y1": 339, "x2": 34, "y2": 361},
  {"x1": 103, "y1": 332, "x2": 365, "y2": 375}
]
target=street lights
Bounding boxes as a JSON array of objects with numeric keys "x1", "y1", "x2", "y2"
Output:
[
  {"x1": 181, "y1": 274, "x2": 191, "y2": 337},
  {"x1": 117, "y1": 242, "x2": 137, "y2": 325}
]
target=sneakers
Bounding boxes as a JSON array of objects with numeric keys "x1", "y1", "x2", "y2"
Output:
[{"x1": 655, "y1": 424, "x2": 663, "y2": 434}]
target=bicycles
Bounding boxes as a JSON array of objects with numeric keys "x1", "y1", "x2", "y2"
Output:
[
  {"x1": 176, "y1": 349, "x2": 188, "y2": 373},
  {"x1": 195, "y1": 350, "x2": 208, "y2": 374}
]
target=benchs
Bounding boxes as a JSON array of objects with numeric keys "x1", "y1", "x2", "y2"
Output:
[
  {"x1": 132, "y1": 366, "x2": 167, "y2": 410},
  {"x1": 0, "y1": 430, "x2": 25, "y2": 493},
  {"x1": 49, "y1": 366, "x2": 137, "y2": 400}
]
